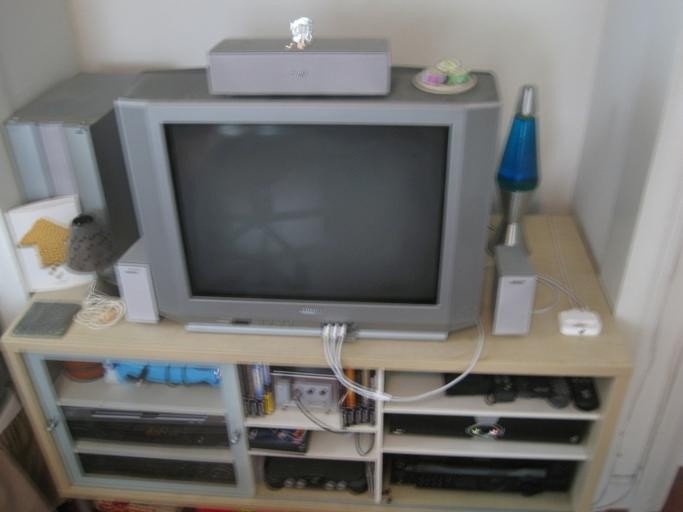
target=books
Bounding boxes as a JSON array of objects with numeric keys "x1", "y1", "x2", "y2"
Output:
[
  {"x1": 5, "y1": 195, "x2": 94, "y2": 290},
  {"x1": 248, "y1": 427, "x2": 312, "y2": 452},
  {"x1": 248, "y1": 367, "x2": 274, "y2": 416},
  {"x1": 341, "y1": 371, "x2": 374, "y2": 428}
]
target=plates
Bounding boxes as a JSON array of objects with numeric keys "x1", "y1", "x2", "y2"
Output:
[{"x1": 411, "y1": 70, "x2": 477, "y2": 95}]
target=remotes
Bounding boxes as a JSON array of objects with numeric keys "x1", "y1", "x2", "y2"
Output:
[
  {"x1": 493, "y1": 375, "x2": 516, "y2": 402},
  {"x1": 547, "y1": 378, "x2": 571, "y2": 406},
  {"x1": 568, "y1": 377, "x2": 599, "y2": 410}
]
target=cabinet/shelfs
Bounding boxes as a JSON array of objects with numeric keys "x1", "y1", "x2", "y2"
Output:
[{"x1": 0, "y1": 212, "x2": 633, "y2": 512}]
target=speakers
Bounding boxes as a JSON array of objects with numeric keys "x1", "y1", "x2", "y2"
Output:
[
  {"x1": 491, "y1": 245, "x2": 537, "y2": 336},
  {"x1": 114, "y1": 237, "x2": 161, "y2": 323},
  {"x1": 207, "y1": 37, "x2": 393, "y2": 96}
]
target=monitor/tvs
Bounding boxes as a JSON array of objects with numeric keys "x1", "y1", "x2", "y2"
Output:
[{"x1": 113, "y1": 66, "x2": 502, "y2": 340}]
